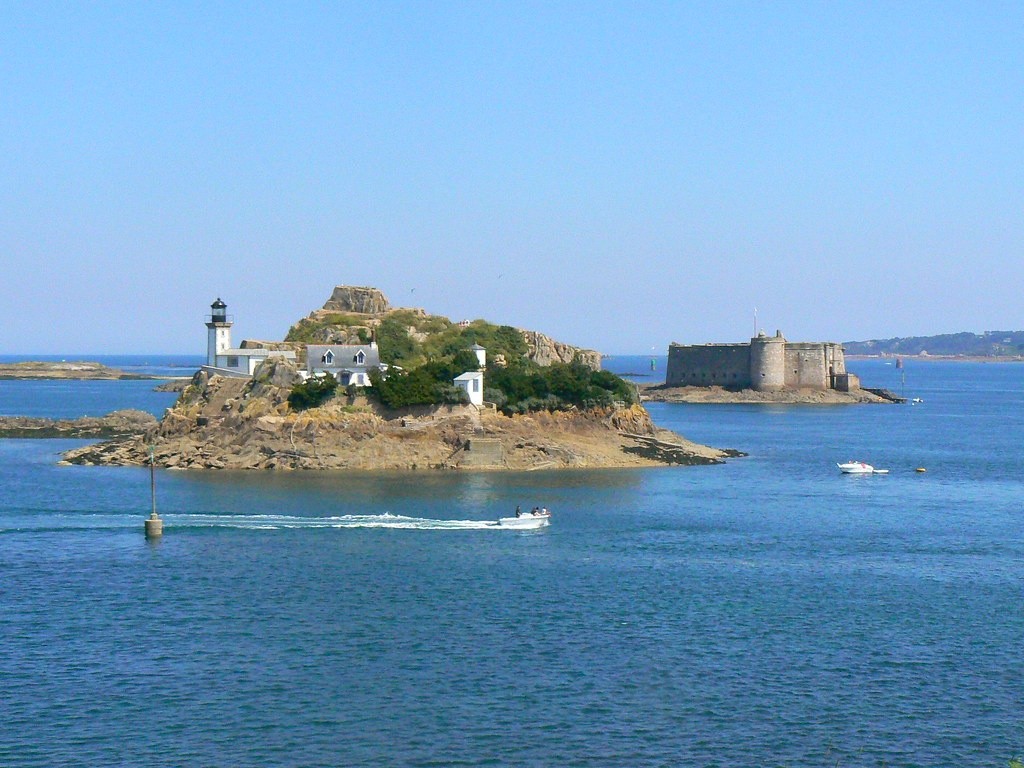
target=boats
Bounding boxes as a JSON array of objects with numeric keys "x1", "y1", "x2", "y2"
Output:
[
  {"x1": 836, "y1": 462, "x2": 873, "y2": 475},
  {"x1": 499, "y1": 513, "x2": 550, "y2": 529},
  {"x1": 912, "y1": 398, "x2": 923, "y2": 403},
  {"x1": 872, "y1": 470, "x2": 889, "y2": 474}
]
